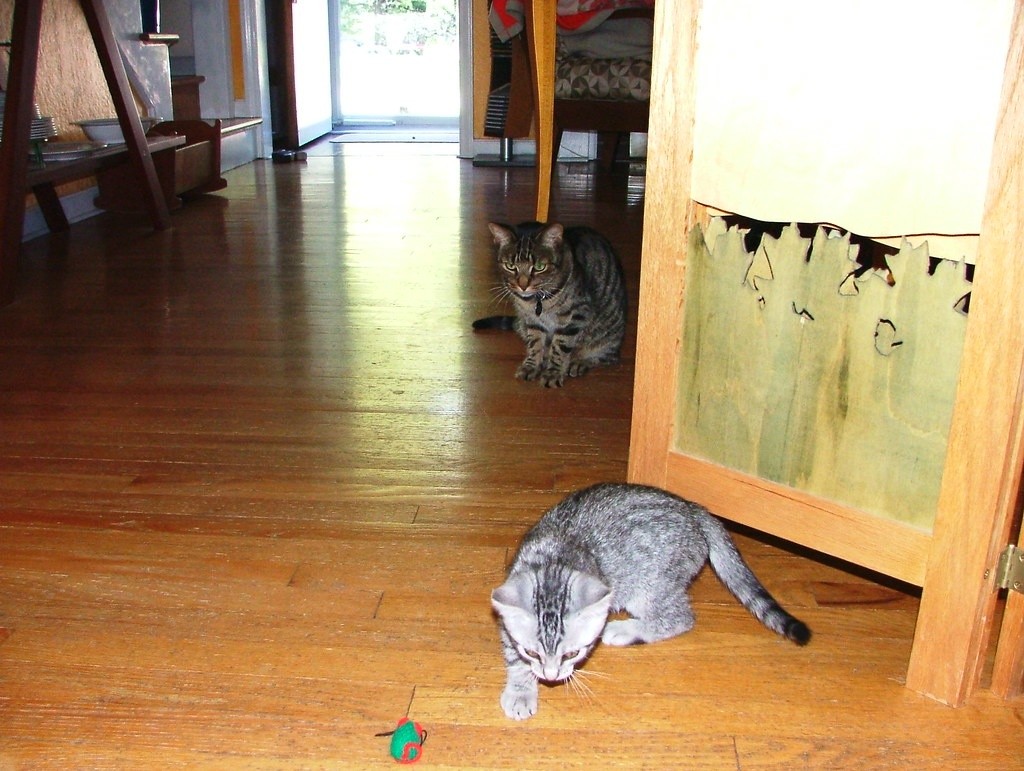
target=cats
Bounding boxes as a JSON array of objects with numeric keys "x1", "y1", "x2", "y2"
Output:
[
  {"x1": 489, "y1": 479, "x2": 811, "y2": 723},
  {"x1": 471, "y1": 216, "x2": 630, "y2": 391}
]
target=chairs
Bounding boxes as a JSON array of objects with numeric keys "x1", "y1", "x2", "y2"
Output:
[{"x1": 501, "y1": 0, "x2": 654, "y2": 223}]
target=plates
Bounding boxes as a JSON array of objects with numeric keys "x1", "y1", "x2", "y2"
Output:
[
  {"x1": 27, "y1": 141, "x2": 108, "y2": 161},
  {"x1": 0, "y1": 91, "x2": 58, "y2": 143}
]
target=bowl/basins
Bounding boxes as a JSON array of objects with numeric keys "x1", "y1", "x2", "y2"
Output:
[{"x1": 69, "y1": 117, "x2": 165, "y2": 144}]
